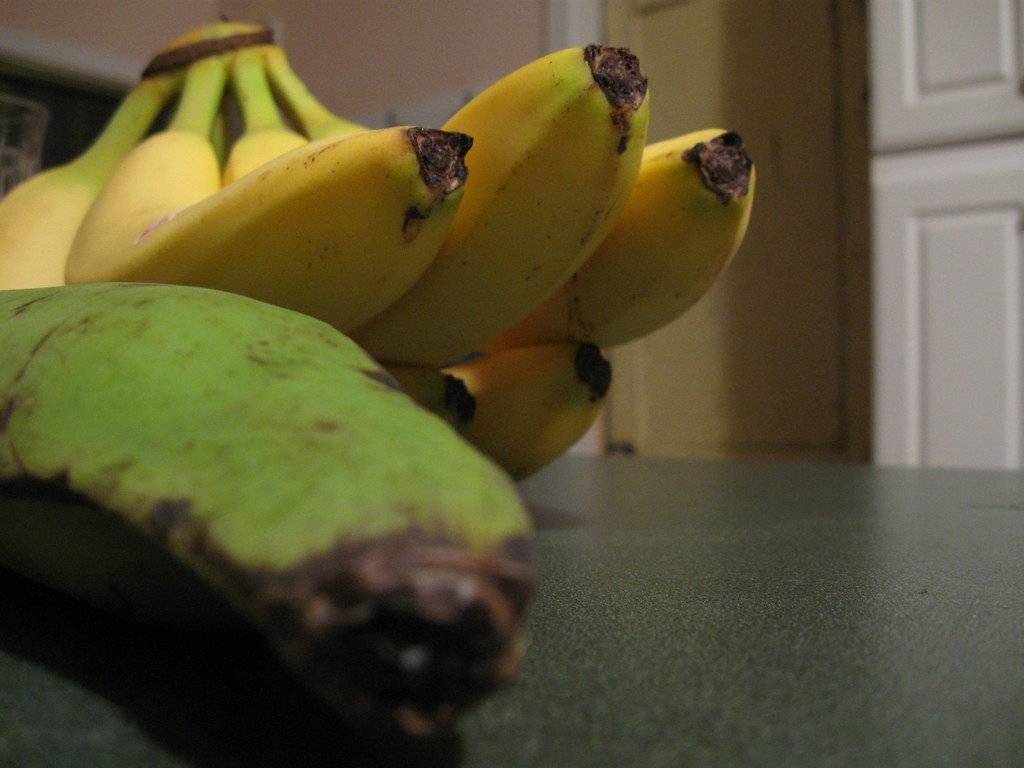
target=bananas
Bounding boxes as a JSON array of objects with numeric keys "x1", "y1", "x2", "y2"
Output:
[{"x1": 0, "y1": 20, "x2": 756, "y2": 472}]
